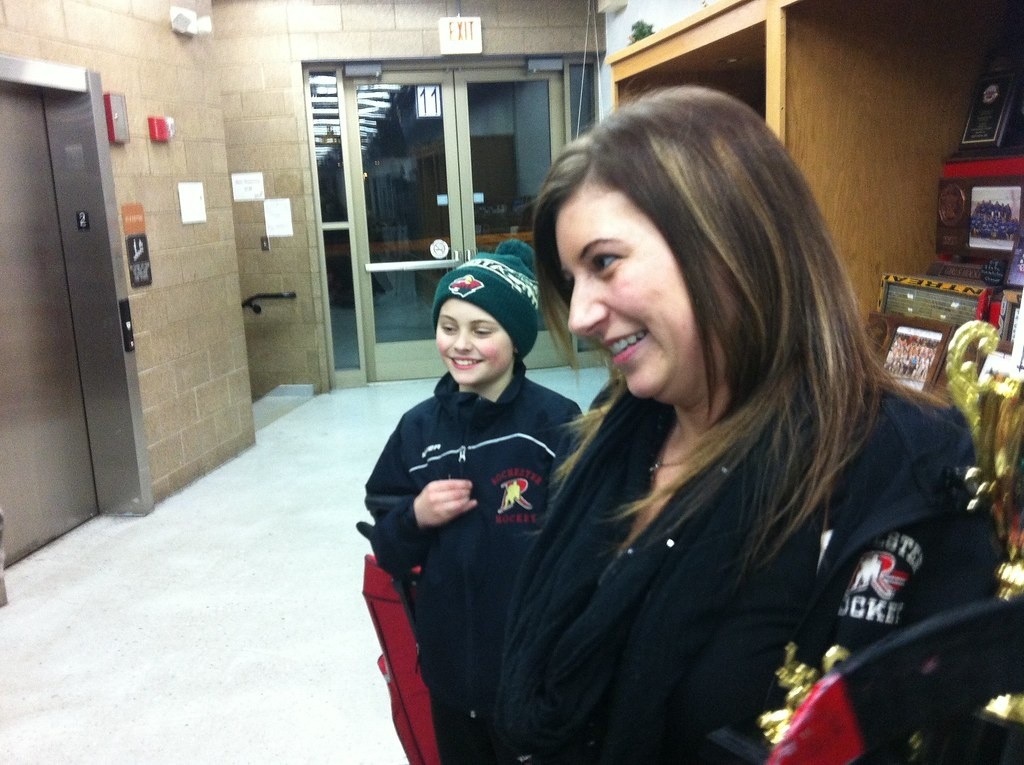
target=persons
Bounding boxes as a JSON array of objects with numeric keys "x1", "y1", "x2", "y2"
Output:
[
  {"x1": 490, "y1": 86, "x2": 1002, "y2": 764},
  {"x1": 969, "y1": 200, "x2": 1019, "y2": 240},
  {"x1": 354, "y1": 239, "x2": 584, "y2": 765},
  {"x1": 883, "y1": 333, "x2": 938, "y2": 383}
]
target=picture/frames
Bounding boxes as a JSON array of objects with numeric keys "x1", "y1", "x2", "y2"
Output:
[
  {"x1": 876, "y1": 317, "x2": 957, "y2": 392},
  {"x1": 958, "y1": 70, "x2": 1016, "y2": 149},
  {"x1": 875, "y1": 272, "x2": 993, "y2": 329}
]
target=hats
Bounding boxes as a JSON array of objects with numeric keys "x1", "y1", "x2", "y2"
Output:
[{"x1": 432, "y1": 239, "x2": 540, "y2": 360}]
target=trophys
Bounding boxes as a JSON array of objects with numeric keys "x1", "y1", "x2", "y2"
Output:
[
  {"x1": 927, "y1": 177, "x2": 984, "y2": 281},
  {"x1": 944, "y1": 318, "x2": 1023, "y2": 601}
]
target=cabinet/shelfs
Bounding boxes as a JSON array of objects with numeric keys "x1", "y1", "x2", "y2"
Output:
[
  {"x1": 604, "y1": 0, "x2": 1024, "y2": 354},
  {"x1": 412, "y1": 134, "x2": 516, "y2": 310}
]
